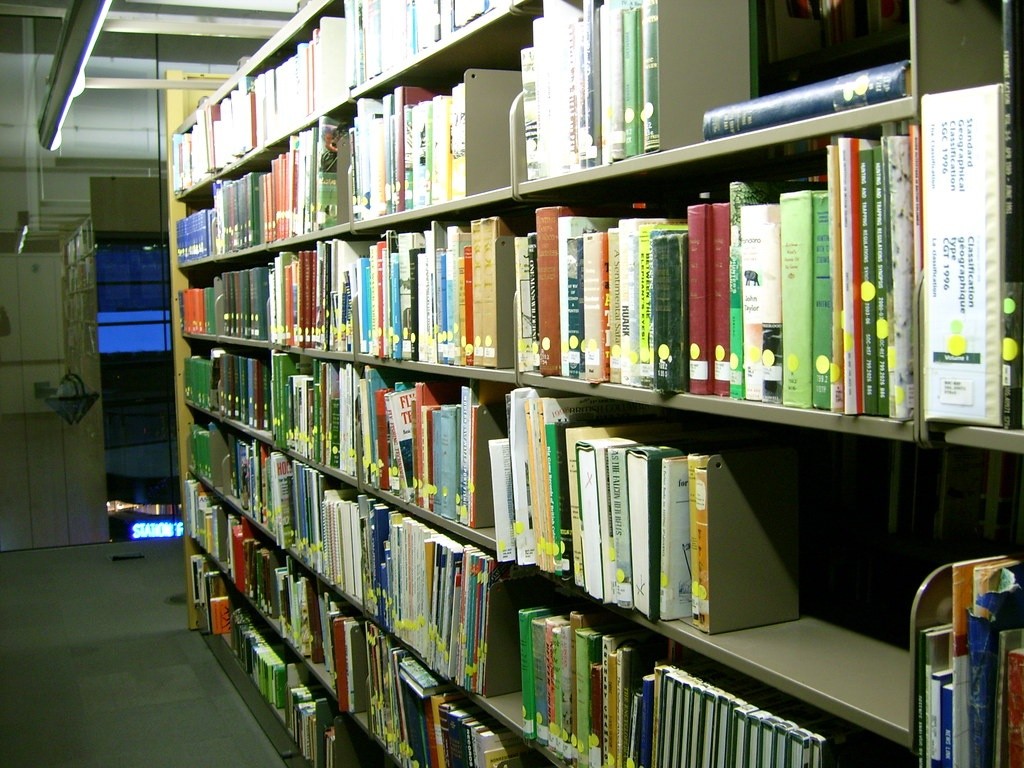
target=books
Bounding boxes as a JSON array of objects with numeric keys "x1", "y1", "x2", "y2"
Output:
[{"x1": 169, "y1": 2, "x2": 1024, "y2": 767}]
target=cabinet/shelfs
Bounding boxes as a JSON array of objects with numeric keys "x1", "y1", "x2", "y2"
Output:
[
  {"x1": 60, "y1": 217, "x2": 102, "y2": 443},
  {"x1": 166, "y1": 0, "x2": 1024, "y2": 768}
]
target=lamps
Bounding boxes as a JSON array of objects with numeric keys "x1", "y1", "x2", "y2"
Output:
[
  {"x1": 14, "y1": 210, "x2": 29, "y2": 253},
  {"x1": 37, "y1": 0, "x2": 112, "y2": 152}
]
